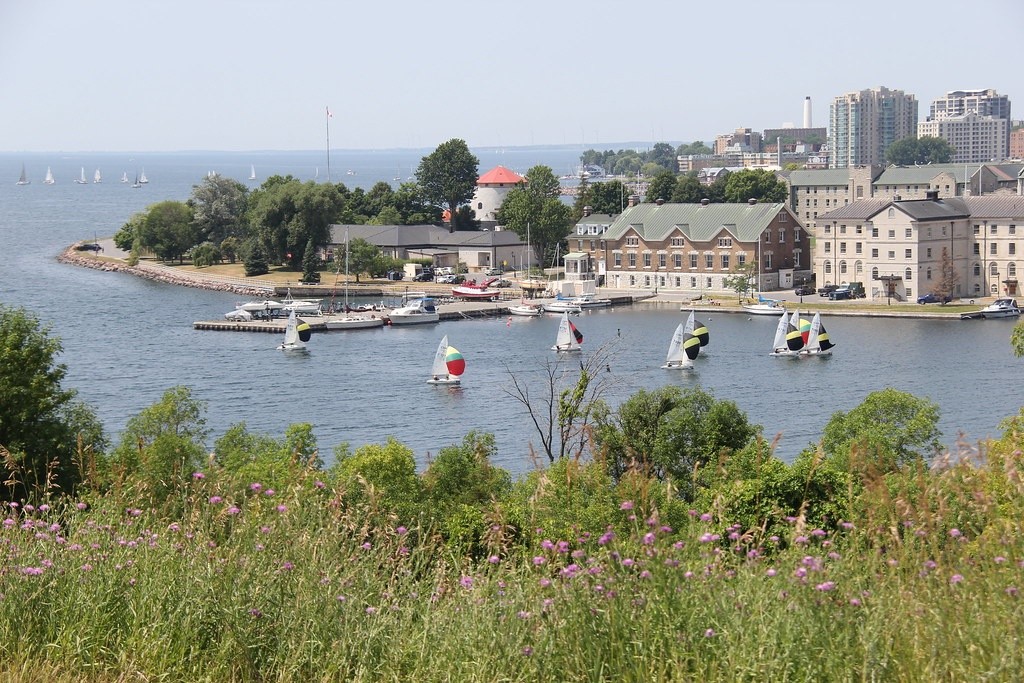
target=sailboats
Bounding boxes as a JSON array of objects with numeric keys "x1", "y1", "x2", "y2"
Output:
[
  {"x1": 660, "y1": 309, "x2": 709, "y2": 370},
  {"x1": 15, "y1": 163, "x2": 31, "y2": 185},
  {"x1": 551, "y1": 308, "x2": 584, "y2": 351},
  {"x1": 425, "y1": 335, "x2": 466, "y2": 384},
  {"x1": 277, "y1": 310, "x2": 311, "y2": 350},
  {"x1": 249, "y1": 164, "x2": 257, "y2": 180},
  {"x1": 94, "y1": 168, "x2": 102, "y2": 184},
  {"x1": 42, "y1": 165, "x2": 55, "y2": 183},
  {"x1": 120, "y1": 165, "x2": 149, "y2": 188},
  {"x1": 769, "y1": 306, "x2": 836, "y2": 356},
  {"x1": 507, "y1": 222, "x2": 611, "y2": 316},
  {"x1": 74, "y1": 166, "x2": 88, "y2": 184},
  {"x1": 740, "y1": 235, "x2": 786, "y2": 315}
]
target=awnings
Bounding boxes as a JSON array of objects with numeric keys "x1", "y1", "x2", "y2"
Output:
[
  {"x1": 1002, "y1": 280, "x2": 1017, "y2": 283},
  {"x1": 875, "y1": 276, "x2": 902, "y2": 280}
]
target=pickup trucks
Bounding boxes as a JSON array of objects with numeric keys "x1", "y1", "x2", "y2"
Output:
[
  {"x1": 917, "y1": 294, "x2": 952, "y2": 305},
  {"x1": 795, "y1": 282, "x2": 866, "y2": 300}
]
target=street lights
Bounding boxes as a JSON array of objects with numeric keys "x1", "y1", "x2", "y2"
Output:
[{"x1": 997, "y1": 272, "x2": 1000, "y2": 298}]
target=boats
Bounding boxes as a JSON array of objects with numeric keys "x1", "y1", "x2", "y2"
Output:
[
  {"x1": 960, "y1": 298, "x2": 1024, "y2": 319},
  {"x1": 225, "y1": 224, "x2": 439, "y2": 330},
  {"x1": 452, "y1": 279, "x2": 500, "y2": 301}
]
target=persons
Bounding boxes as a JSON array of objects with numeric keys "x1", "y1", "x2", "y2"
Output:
[
  {"x1": 710, "y1": 299, "x2": 714, "y2": 303},
  {"x1": 606, "y1": 364, "x2": 611, "y2": 372},
  {"x1": 282, "y1": 342, "x2": 285, "y2": 348},
  {"x1": 557, "y1": 345, "x2": 561, "y2": 349},
  {"x1": 617, "y1": 329, "x2": 621, "y2": 337},
  {"x1": 580, "y1": 361, "x2": 583, "y2": 370},
  {"x1": 667, "y1": 362, "x2": 672, "y2": 367},
  {"x1": 434, "y1": 375, "x2": 439, "y2": 380}
]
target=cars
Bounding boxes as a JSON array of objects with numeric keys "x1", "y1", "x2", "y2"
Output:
[
  {"x1": 411, "y1": 266, "x2": 467, "y2": 284},
  {"x1": 481, "y1": 276, "x2": 512, "y2": 287},
  {"x1": 485, "y1": 268, "x2": 504, "y2": 275}
]
